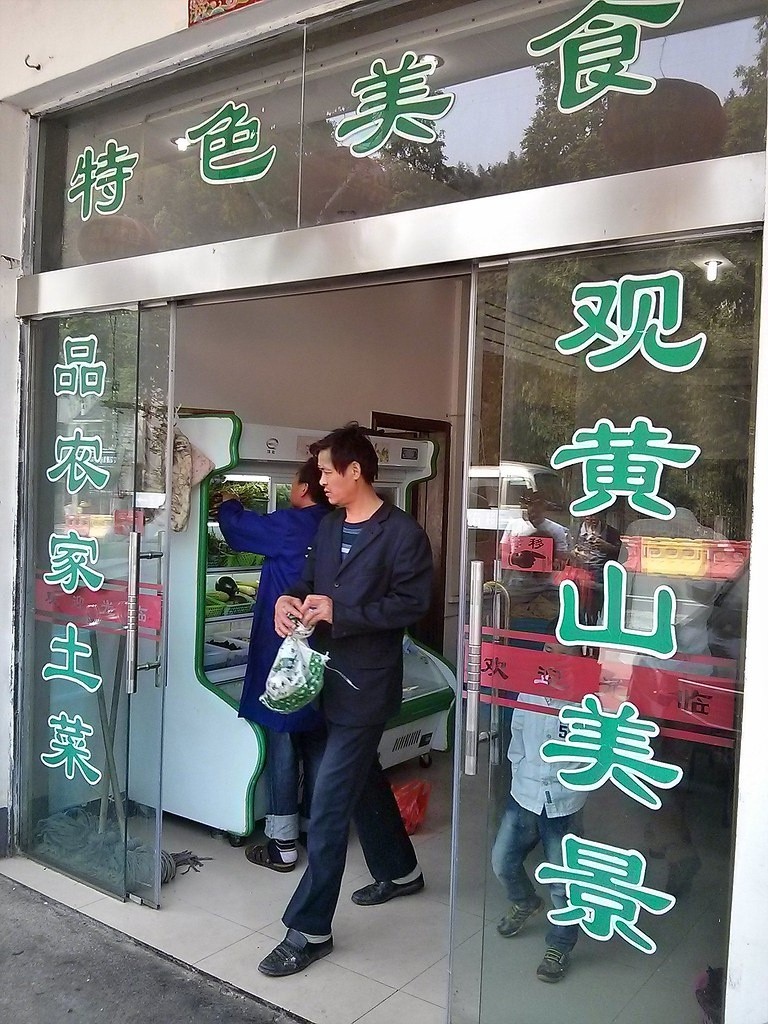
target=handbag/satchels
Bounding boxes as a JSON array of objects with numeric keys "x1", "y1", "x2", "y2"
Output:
[
  {"x1": 390, "y1": 779, "x2": 431, "y2": 835},
  {"x1": 259, "y1": 607, "x2": 332, "y2": 715},
  {"x1": 552, "y1": 548, "x2": 596, "y2": 626}
]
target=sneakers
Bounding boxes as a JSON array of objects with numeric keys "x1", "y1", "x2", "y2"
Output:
[
  {"x1": 497, "y1": 890, "x2": 545, "y2": 938},
  {"x1": 536, "y1": 946, "x2": 577, "y2": 982}
]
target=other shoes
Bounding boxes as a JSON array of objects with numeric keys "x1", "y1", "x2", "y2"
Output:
[
  {"x1": 666, "y1": 847, "x2": 698, "y2": 905},
  {"x1": 643, "y1": 822, "x2": 666, "y2": 859}
]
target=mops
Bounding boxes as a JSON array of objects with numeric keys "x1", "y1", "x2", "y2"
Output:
[{"x1": 35, "y1": 607, "x2": 213, "y2": 892}]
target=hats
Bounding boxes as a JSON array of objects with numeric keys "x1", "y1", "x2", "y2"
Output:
[{"x1": 518, "y1": 486, "x2": 545, "y2": 504}]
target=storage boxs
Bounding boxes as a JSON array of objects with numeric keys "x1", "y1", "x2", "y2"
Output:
[
  {"x1": 216, "y1": 629, "x2": 251, "y2": 644},
  {"x1": 205, "y1": 634, "x2": 249, "y2": 667},
  {"x1": 203, "y1": 643, "x2": 229, "y2": 672}
]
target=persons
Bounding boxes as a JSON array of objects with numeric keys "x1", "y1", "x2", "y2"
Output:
[
  {"x1": 488, "y1": 477, "x2": 747, "y2": 983},
  {"x1": 215, "y1": 456, "x2": 335, "y2": 873},
  {"x1": 257, "y1": 422, "x2": 433, "y2": 978}
]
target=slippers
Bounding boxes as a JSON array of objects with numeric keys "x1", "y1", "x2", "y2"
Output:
[
  {"x1": 297, "y1": 832, "x2": 307, "y2": 847},
  {"x1": 244, "y1": 845, "x2": 295, "y2": 872}
]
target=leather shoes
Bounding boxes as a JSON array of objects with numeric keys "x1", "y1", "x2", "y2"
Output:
[
  {"x1": 258, "y1": 928, "x2": 334, "y2": 977},
  {"x1": 351, "y1": 872, "x2": 426, "y2": 906}
]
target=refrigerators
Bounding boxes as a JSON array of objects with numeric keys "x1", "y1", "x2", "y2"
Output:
[{"x1": 129, "y1": 415, "x2": 467, "y2": 848}]
